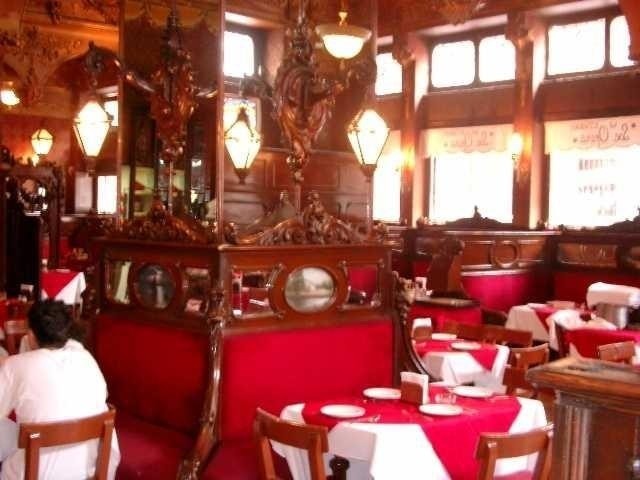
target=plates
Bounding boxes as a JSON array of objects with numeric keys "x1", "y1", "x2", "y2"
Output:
[
  {"x1": 418, "y1": 401, "x2": 463, "y2": 418},
  {"x1": 451, "y1": 384, "x2": 493, "y2": 400},
  {"x1": 364, "y1": 386, "x2": 402, "y2": 401},
  {"x1": 320, "y1": 405, "x2": 367, "y2": 418}
]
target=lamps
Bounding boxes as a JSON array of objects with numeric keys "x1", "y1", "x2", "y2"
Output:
[
  {"x1": 223, "y1": 76, "x2": 265, "y2": 185},
  {"x1": 31, "y1": 115, "x2": 54, "y2": 161},
  {"x1": 315, "y1": 0, "x2": 372, "y2": 61},
  {"x1": 345, "y1": 102, "x2": 392, "y2": 245},
  {"x1": 0, "y1": 67, "x2": 22, "y2": 108},
  {"x1": 70, "y1": 64, "x2": 112, "y2": 172}
]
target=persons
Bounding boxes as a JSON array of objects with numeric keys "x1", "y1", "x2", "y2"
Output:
[{"x1": 0, "y1": 299, "x2": 122, "y2": 480}]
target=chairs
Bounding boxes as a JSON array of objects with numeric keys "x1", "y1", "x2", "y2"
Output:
[
  {"x1": 503, "y1": 343, "x2": 551, "y2": 402},
  {"x1": 452, "y1": 322, "x2": 490, "y2": 342},
  {"x1": 253, "y1": 408, "x2": 331, "y2": 480},
  {"x1": 596, "y1": 342, "x2": 636, "y2": 369},
  {"x1": 474, "y1": 423, "x2": 557, "y2": 479},
  {"x1": 19, "y1": 409, "x2": 115, "y2": 480},
  {"x1": 486, "y1": 326, "x2": 534, "y2": 369}
]
